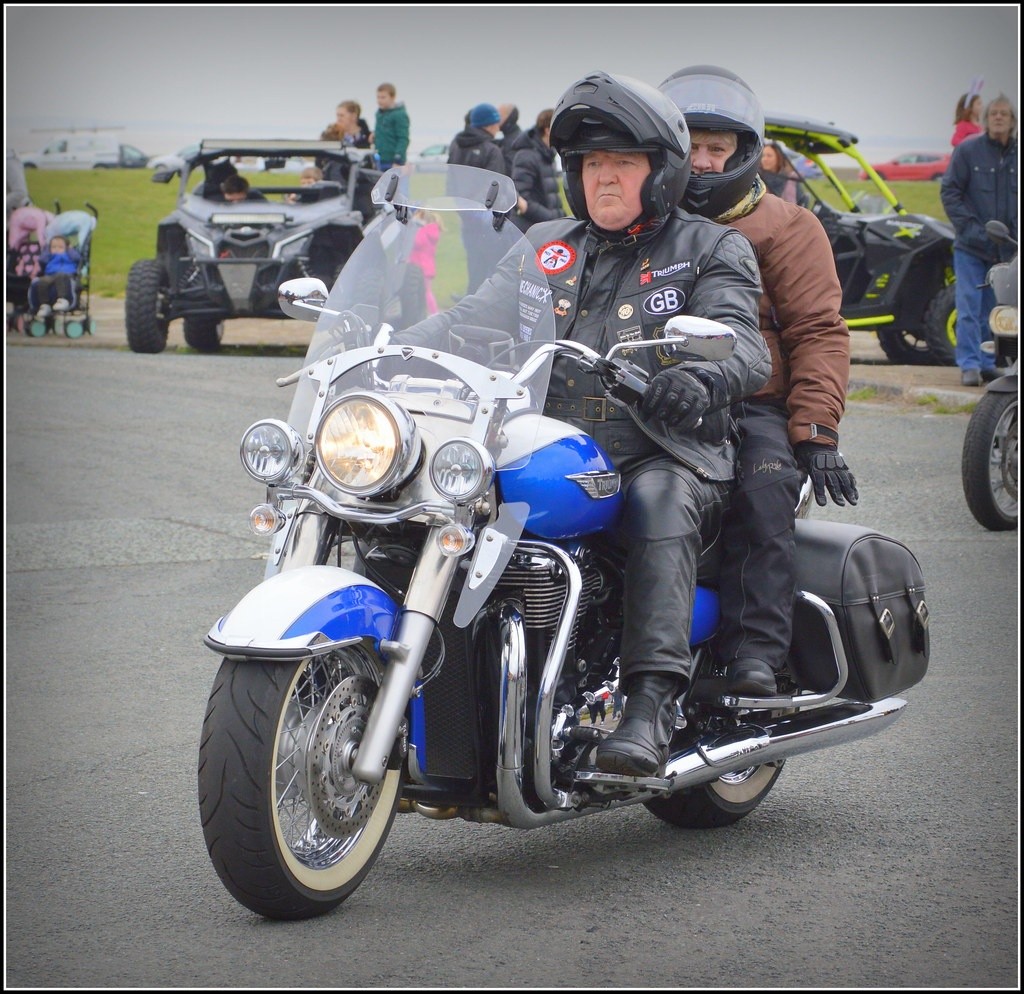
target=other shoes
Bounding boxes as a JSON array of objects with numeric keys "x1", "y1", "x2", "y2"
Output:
[
  {"x1": 36, "y1": 304, "x2": 52, "y2": 317},
  {"x1": 961, "y1": 370, "x2": 981, "y2": 387},
  {"x1": 53, "y1": 298, "x2": 70, "y2": 311},
  {"x1": 980, "y1": 366, "x2": 1006, "y2": 381}
]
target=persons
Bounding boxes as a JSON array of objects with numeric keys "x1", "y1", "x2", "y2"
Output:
[
  {"x1": 661, "y1": 67, "x2": 859, "y2": 699},
  {"x1": 411, "y1": 207, "x2": 444, "y2": 316},
  {"x1": 32, "y1": 236, "x2": 80, "y2": 317},
  {"x1": 312, "y1": 68, "x2": 773, "y2": 774},
  {"x1": 368, "y1": 83, "x2": 410, "y2": 174},
  {"x1": 447, "y1": 103, "x2": 566, "y2": 293},
  {"x1": 288, "y1": 101, "x2": 381, "y2": 218},
  {"x1": 939, "y1": 97, "x2": 1018, "y2": 386},
  {"x1": 210, "y1": 175, "x2": 267, "y2": 202},
  {"x1": 6, "y1": 149, "x2": 31, "y2": 264},
  {"x1": 951, "y1": 93, "x2": 984, "y2": 146},
  {"x1": 760, "y1": 144, "x2": 796, "y2": 204}
]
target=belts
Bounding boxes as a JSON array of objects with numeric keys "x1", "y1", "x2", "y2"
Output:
[{"x1": 536, "y1": 395, "x2": 632, "y2": 422}]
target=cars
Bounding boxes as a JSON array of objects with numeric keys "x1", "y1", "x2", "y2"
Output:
[
  {"x1": 783, "y1": 150, "x2": 823, "y2": 180},
  {"x1": 107, "y1": 145, "x2": 150, "y2": 169},
  {"x1": 407, "y1": 143, "x2": 451, "y2": 172},
  {"x1": 859, "y1": 152, "x2": 951, "y2": 183},
  {"x1": 146, "y1": 143, "x2": 315, "y2": 173}
]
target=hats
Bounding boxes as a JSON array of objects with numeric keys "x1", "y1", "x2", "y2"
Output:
[
  {"x1": 470, "y1": 103, "x2": 500, "y2": 130},
  {"x1": 302, "y1": 167, "x2": 321, "y2": 182},
  {"x1": 221, "y1": 175, "x2": 248, "y2": 193}
]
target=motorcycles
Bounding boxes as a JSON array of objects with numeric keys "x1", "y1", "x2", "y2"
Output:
[
  {"x1": 197, "y1": 160, "x2": 933, "y2": 923},
  {"x1": 961, "y1": 220, "x2": 1018, "y2": 533}
]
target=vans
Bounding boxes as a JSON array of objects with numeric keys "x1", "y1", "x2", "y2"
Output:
[{"x1": 18, "y1": 133, "x2": 121, "y2": 171}]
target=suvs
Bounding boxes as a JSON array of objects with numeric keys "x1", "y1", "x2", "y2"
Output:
[
  {"x1": 759, "y1": 118, "x2": 958, "y2": 367},
  {"x1": 124, "y1": 139, "x2": 428, "y2": 355}
]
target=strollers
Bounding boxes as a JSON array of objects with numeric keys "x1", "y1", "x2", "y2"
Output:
[
  {"x1": 6, "y1": 201, "x2": 54, "y2": 334},
  {"x1": 24, "y1": 201, "x2": 99, "y2": 339}
]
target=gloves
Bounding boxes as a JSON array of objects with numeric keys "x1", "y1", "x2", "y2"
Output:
[
  {"x1": 350, "y1": 316, "x2": 397, "y2": 384},
  {"x1": 793, "y1": 442, "x2": 862, "y2": 508},
  {"x1": 638, "y1": 364, "x2": 710, "y2": 438}
]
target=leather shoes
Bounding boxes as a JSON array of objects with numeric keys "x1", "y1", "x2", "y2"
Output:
[
  {"x1": 598, "y1": 671, "x2": 681, "y2": 780},
  {"x1": 730, "y1": 656, "x2": 779, "y2": 698}
]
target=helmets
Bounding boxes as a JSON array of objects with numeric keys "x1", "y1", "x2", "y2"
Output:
[
  {"x1": 657, "y1": 64, "x2": 766, "y2": 221},
  {"x1": 546, "y1": 71, "x2": 692, "y2": 252}
]
